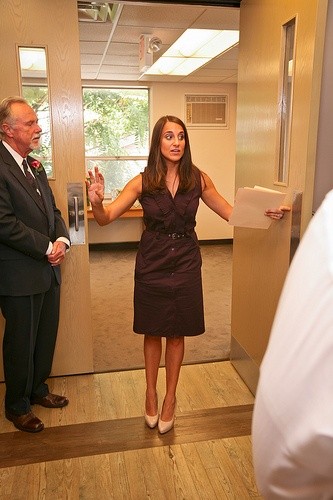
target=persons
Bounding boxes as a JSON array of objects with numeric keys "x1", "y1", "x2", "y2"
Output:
[
  {"x1": 0, "y1": 95, "x2": 71, "y2": 432},
  {"x1": 251, "y1": 188, "x2": 333, "y2": 500},
  {"x1": 84, "y1": 115, "x2": 290, "y2": 434}
]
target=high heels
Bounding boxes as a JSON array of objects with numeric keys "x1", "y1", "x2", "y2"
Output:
[{"x1": 144, "y1": 394, "x2": 177, "y2": 434}]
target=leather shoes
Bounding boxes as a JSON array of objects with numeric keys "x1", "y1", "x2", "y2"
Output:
[
  {"x1": 5, "y1": 410, "x2": 44, "y2": 433},
  {"x1": 30, "y1": 393, "x2": 70, "y2": 408}
]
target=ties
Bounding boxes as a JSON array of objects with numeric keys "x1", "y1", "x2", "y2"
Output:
[{"x1": 21, "y1": 160, "x2": 40, "y2": 191}]
